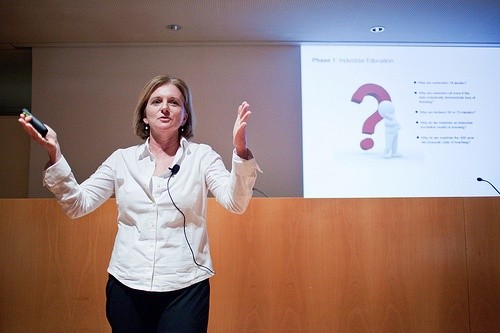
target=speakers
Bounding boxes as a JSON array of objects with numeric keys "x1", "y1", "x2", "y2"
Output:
[{"x1": 1, "y1": 49, "x2": 32, "y2": 116}]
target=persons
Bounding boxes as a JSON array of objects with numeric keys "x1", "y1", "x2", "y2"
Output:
[
  {"x1": 378, "y1": 101, "x2": 404, "y2": 159},
  {"x1": 16, "y1": 72, "x2": 262, "y2": 333}
]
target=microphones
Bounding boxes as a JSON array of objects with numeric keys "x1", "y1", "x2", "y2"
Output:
[
  {"x1": 169, "y1": 164, "x2": 180, "y2": 175},
  {"x1": 252, "y1": 187, "x2": 268, "y2": 197},
  {"x1": 477, "y1": 178, "x2": 500, "y2": 194}
]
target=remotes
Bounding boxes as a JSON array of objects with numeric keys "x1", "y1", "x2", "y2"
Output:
[{"x1": 22, "y1": 108, "x2": 48, "y2": 137}]
